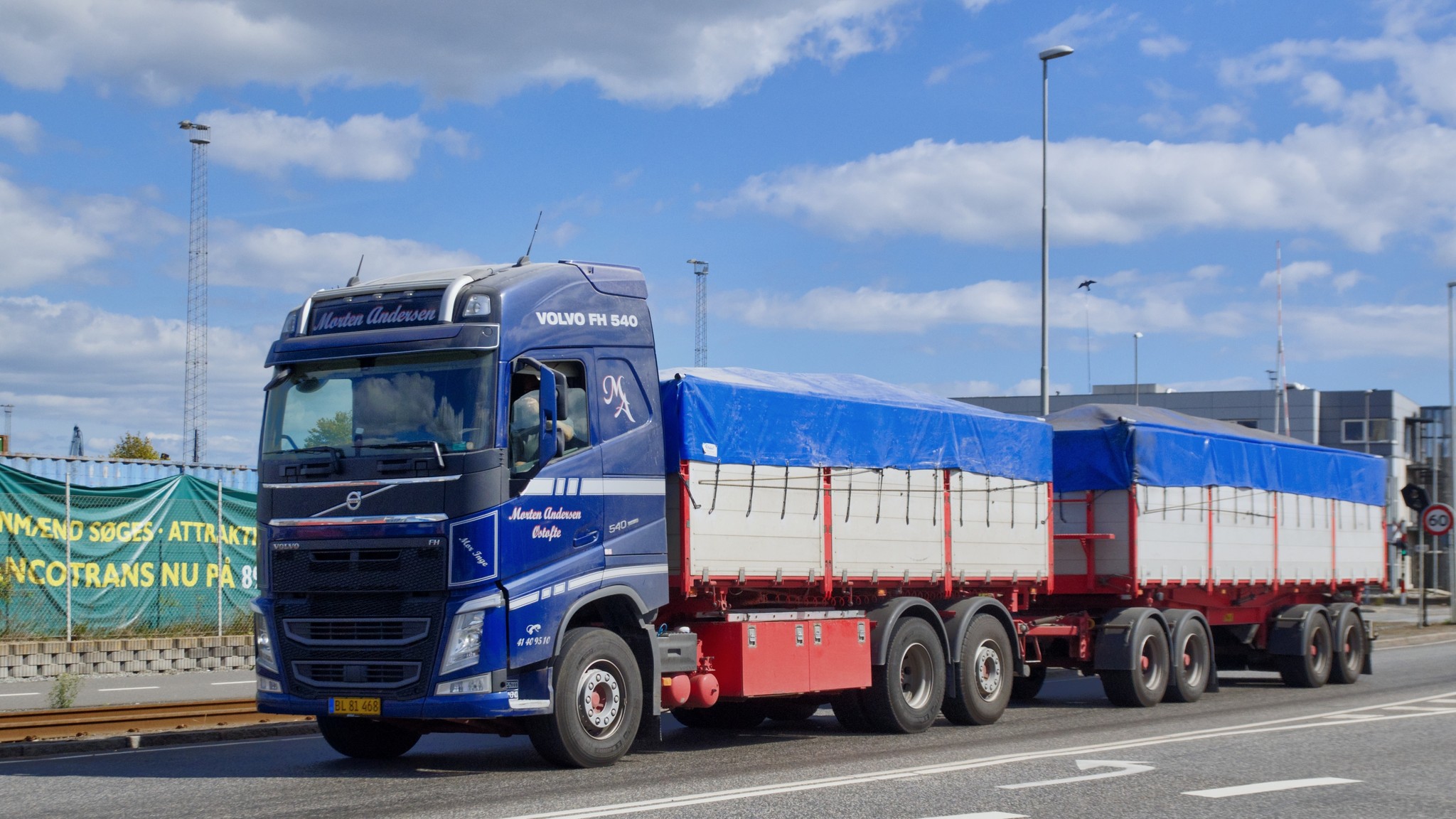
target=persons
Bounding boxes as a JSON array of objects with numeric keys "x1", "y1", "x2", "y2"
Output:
[{"x1": 513, "y1": 361, "x2": 588, "y2": 450}]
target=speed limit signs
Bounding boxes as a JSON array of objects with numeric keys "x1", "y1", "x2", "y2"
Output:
[{"x1": 1422, "y1": 503, "x2": 1454, "y2": 536}]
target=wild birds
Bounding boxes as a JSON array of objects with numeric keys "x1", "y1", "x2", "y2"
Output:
[{"x1": 1077, "y1": 279, "x2": 1098, "y2": 291}]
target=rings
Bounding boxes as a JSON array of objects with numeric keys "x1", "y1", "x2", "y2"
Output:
[{"x1": 529, "y1": 405, "x2": 530, "y2": 408}]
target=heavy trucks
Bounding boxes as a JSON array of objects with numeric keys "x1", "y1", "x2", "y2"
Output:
[{"x1": 248, "y1": 259, "x2": 1390, "y2": 773}]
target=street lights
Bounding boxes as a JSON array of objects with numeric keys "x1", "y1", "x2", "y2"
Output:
[
  {"x1": 1036, "y1": 45, "x2": 1077, "y2": 418},
  {"x1": 1133, "y1": 331, "x2": 1144, "y2": 406}
]
